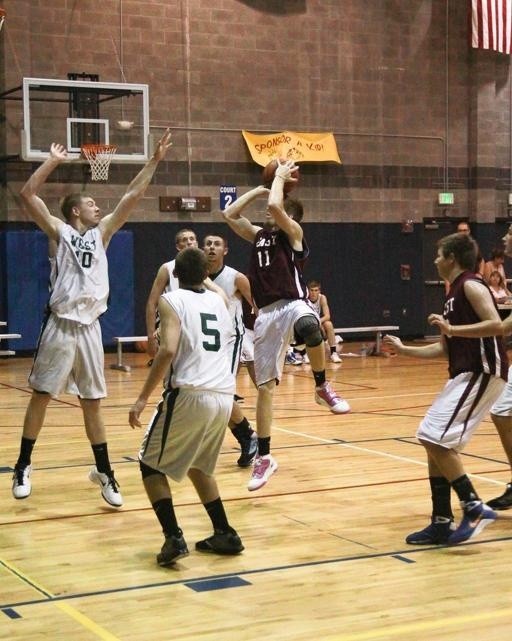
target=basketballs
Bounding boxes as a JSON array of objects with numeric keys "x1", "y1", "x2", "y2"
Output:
[
  {"x1": 262, "y1": 158, "x2": 300, "y2": 193},
  {"x1": 135, "y1": 340, "x2": 149, "y2": 353}
]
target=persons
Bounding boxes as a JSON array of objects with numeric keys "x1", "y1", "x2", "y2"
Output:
[
  {"x1": 129, "y1": 159, "x2": 351, "y2": 566},
  {"x1": 11, "y1": 126, "x2": 172, "y2": 507},
  {"x1": 380, "y1": 221, "x2": 512, "y2": 546}
]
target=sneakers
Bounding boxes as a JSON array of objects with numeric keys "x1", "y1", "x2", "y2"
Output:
[
  {"x1": 405, "y1": 481, "x2": 512, "y2": 547},
  {"x1": 195, "y1": 527, "x2": 245, "y2": 556},
  {"x1": 314, "y1": 380, "x2": 352, "y2": 415},
  {"x1": 237, "y1": 430, "x2": 259, "y2": 468},
  {"x1": 156, "y1": 534, "x2": 190, "y2": 566},
  {"x1": 246, "y1": 453, "x2": 280, "y2": 493},
  {"x1": 87, "y1": 463, "x2": 125, "y2": 508},
  {"x1": 11, "y1": 461, "x2": 34, "y2": 499},
  {"x1": 329, "y1": 352, "x2": 343, "y2": 363},
  {"x1": 284, "y1": 351, "x2": 311, "y2": 366}
]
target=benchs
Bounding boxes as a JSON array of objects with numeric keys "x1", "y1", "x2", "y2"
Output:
[
  {"x1": 107, "y1": 335, "x2": 149, "y2": 372},
  {"x1": 332, "y1": 324, "x2": 401, "y2": 356},
  {"x1": 0, "y1": 318, "x2": 23, "y2": 357}
]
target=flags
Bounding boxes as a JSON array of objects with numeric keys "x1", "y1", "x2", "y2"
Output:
[{"x1": 470, "y1": 1, "x2": 511, "y2": 58}]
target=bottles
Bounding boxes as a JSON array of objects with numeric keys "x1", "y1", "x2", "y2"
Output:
[{"x1": 360, "y1": 344, "x2": 366, "y2": 357}]
